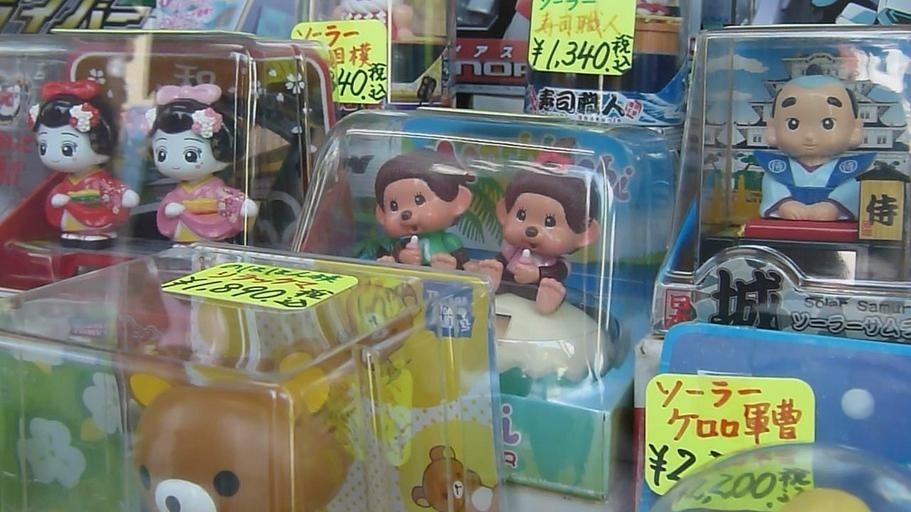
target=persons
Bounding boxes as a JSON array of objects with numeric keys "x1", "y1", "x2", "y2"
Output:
[
  {"x1": 137, "y1": 83, "x2": 260, "y2": 246},
  {"x1": 751, "y1": 62, "x2": 878, "y2": 223},
  {"x1": 353, "y1": 146, "x2": 473, "y2": 271},
  {"x1": 479, "y1": 136, "x2": 603, "y2": 315},
  {"x1": 26, "y1": 78, "x2": 138, "y2": 249}
]
